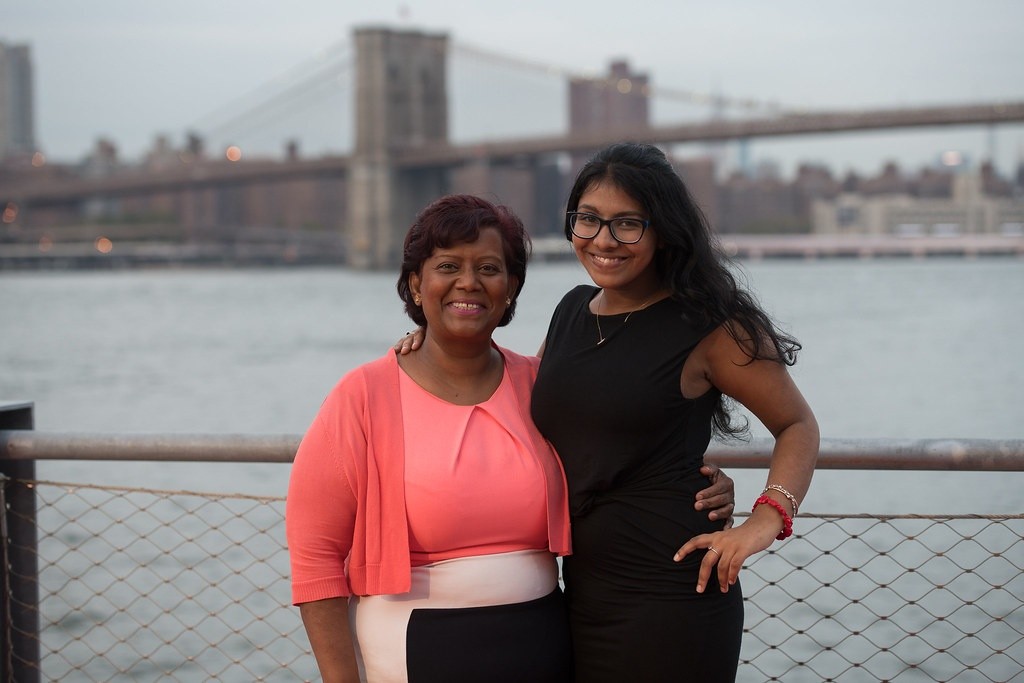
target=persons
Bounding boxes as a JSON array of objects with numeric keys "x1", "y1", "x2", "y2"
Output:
[{"x1": 285, "y1": 140, "x2": 821, "y2": 683}]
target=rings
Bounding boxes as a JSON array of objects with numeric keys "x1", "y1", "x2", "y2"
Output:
[
  {"x1": 405, "y1": 330, "x2": 416, "y2": 337},
  {"x1": 707, "y1": 545, "x2": 721, "y2": 557}
]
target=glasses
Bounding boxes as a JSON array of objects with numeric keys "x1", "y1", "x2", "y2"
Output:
[{"x1": 566, "y1": 211, "x2": 652, "y2": 244}]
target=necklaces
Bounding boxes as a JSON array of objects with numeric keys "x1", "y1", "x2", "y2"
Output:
[{"x1": 596, "y1": 288, "x2": 655, "y2": 346}]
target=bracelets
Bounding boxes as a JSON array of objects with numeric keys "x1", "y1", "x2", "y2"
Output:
[
  {"x1": 751, "y1": 495, "x2": 793, "y2": 542},
  {"x1": 760, "y1": 484, "x2": 799, "y2": 519}
]
